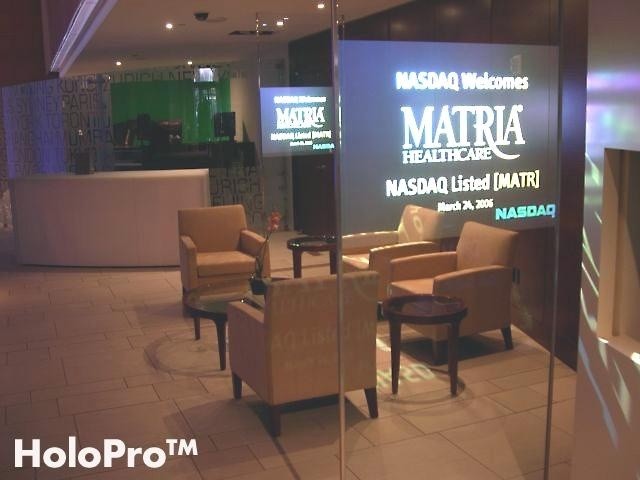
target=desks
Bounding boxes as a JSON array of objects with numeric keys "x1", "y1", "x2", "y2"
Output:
[
  {"x1": 381, "y1": 293, "x2": 475, "y2": 396},
  {"x1": 287, "y1": 235, "x2": 337, "y2": 278}
]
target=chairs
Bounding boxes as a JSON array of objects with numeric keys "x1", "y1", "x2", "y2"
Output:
[
  {"x1": 176, "y1": 204, "x2": 271, "y2": 314},
  {"x1": 342, "y1": 204, "x2": 522, "y2": 363},
  {"x1": 223, "y1": 270, "x2": 385, "y2": 438}
]
